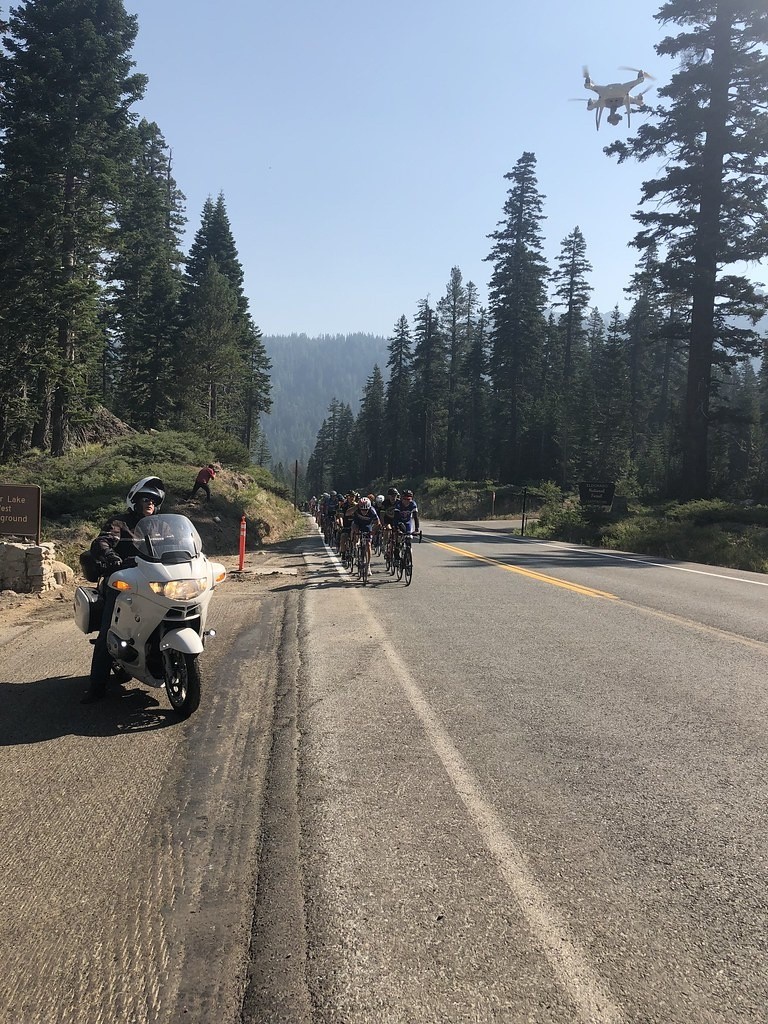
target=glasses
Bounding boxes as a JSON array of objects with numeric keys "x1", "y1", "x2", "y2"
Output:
[{"x1": 136, "y1": 497, "x2": 157, "y2": 507}]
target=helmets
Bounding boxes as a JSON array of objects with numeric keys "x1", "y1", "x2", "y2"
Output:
[
  {"x1": 346, "y1": 490, "x2": 356, "y2": 497},
  {"x1": 359, "y1": 497, "x2": 372, "y2": 510},
  {"x1": 387, "y1": 487, "x2": 398, "y2": 495},
  {"x1": 313, "y1": 491, "x2": 344, "y2": 500},
  {"x1": 401, "y1": 489, "x2": 413, "y2": 498},
  {"x1": 126, "y1": 475, "x2": 166, "y2": 516},
  {"x1": 376, "y1": 495, "x2": 385, "y2": 502}
]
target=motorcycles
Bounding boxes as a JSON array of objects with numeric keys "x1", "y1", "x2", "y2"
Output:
[{"x1": 73, "y1": 517, "x2": 225, "y2": 718}]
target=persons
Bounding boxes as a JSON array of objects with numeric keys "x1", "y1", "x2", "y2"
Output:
[
  {"x1": 301, "y1": 488, "x2": 420, "y2": 576},
  {"x1": 78, "y1": 476, "x2": 193, "y2": 704},
  {"x1": 188, "y1": 464, "x2": 216, "y2": 501}
]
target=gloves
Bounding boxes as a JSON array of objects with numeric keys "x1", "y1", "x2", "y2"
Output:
[{"x1": 105, "y1": 554, "x2": 123, "y2": 573}]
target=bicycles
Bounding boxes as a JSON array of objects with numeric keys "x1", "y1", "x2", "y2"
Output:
[{"x1": 313, "y1": 511, "x2": 424, "y2": 587}]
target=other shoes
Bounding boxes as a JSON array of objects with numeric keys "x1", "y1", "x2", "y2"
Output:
[
  {"x1": 365, "y1": 566, "x2": 372, "y2": 576},
  {"x1": 79, "y1": 690, "x2": 105, "y2": 704}
]
